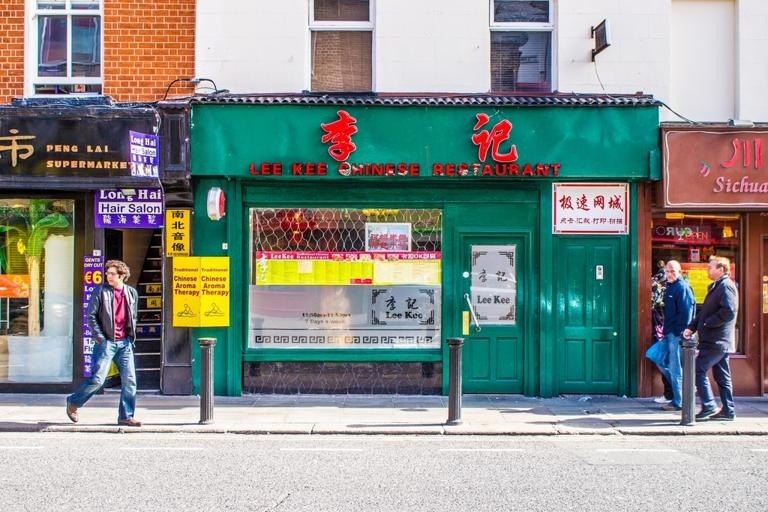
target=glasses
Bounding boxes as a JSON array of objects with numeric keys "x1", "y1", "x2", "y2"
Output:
[{"x1": 105, "y1": 272, "x2": 118, "y2": 275}]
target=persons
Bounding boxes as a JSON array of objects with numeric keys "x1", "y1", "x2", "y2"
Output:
[
  {"x1": 653, "y1": 373, "x2": 674, "y2": 404},
  {"x1": 65, "y1": 260, "x2": 143, "y2": 427},
  {"x1": 645, "y1": 259, "x2": 697, "y2": 411},
  {"x1": 683, "y1": 255, "x2": 740, "y2": 421}
]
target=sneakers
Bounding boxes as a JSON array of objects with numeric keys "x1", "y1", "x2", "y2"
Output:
[
  {"x1": 710, "y1": 409, "x2": 736, "y2": 421},
  {"x1": 695, "y1": 406, "x2": 718, "y2": 421},
  {"x1": 654, "y1": 396, "x2": 680, "y2": 410}
]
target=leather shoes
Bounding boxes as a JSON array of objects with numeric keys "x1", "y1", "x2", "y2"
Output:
[
  {"x1": 118, "y1": 419, "x2": 141, "y2": 426},
  {"x1": 67, "y1": 399, "x2": 79, "y2": 422}
]
